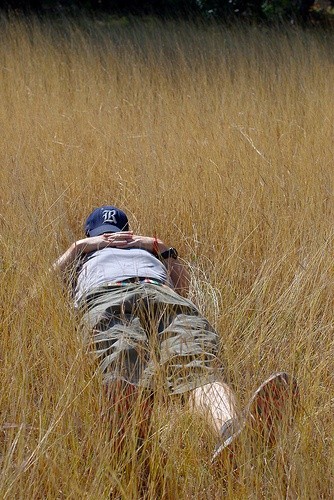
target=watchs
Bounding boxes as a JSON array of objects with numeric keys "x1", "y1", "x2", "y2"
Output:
[{"x1": 161, "y1": 246, "x2": 178, "y2": 260}]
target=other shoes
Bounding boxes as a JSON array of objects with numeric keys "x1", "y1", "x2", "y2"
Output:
[{"x1": 212, "y1": 371, "x2": 299, "y2": 474}]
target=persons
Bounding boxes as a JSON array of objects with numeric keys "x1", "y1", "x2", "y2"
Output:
[{"x1": 30, "y1": 205, "x2": 302, "y2": 500}]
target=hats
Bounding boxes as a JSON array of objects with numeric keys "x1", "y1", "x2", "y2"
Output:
[{"x1": 84, "y1": 205, "x2": 129, "y2": 237}]
